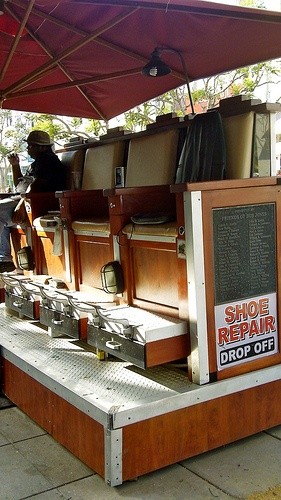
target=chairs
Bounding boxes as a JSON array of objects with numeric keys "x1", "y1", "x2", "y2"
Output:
[
  {"x1": 103, "y1": 93, "x2": 280, "y2": 323},
  {"x1": 55, "y1": 112, "x2": 187, "y2": 306},
  {"x1": 0, "y1": 144, "x2": 87, "y2": 276},
  {"x1": 21, "y1": 127, "x2": 133, "y2": 291}
]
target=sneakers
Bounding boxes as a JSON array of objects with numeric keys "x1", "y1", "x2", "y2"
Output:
[{"x1": 0, "y1": 262, "x2": 16, "y2": 273}]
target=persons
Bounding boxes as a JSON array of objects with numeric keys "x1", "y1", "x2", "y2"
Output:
[{"x1": 6, "y1": 130, "x2": 67, "y2": 192}]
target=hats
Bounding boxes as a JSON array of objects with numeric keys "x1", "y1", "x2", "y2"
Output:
[{"x1": 23, "y1": 130, "x2": 53, "y2": 146}]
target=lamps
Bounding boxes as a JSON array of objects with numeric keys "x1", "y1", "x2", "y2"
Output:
[{"x1": 142, "y1": 47, "x2": 196, "y2": 116}]
